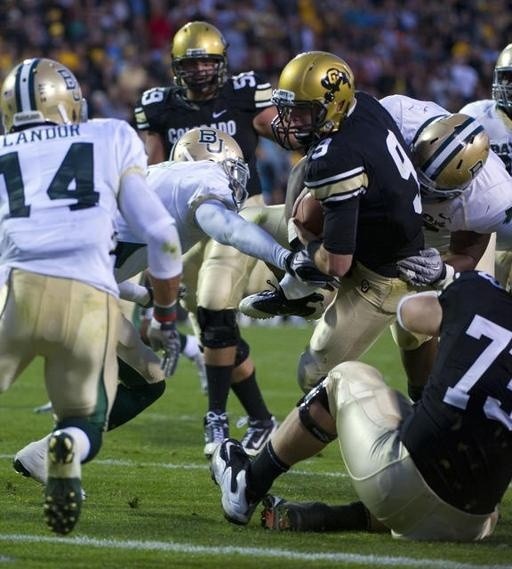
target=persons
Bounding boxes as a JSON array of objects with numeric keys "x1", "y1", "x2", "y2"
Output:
[
  {"x1": 209, "y1": 269, "x2": 512, "y2": 538},
  {"x1": 256, "y1": 136, "x2": 303, "y2": 205},
  {"x1": 0, "y1": 0, "x2": 172, "y2": 124},
  {"x1": 134, "y1": 21, "x2": 281, "y2": 458},
  {"x1": 14, "y1": 126, "x2": 341, "y2": 499},
  {"x1": 279, "y1": 0, "x2": 512, "y2": 113},
  {"x1": 1, "y1": 56, "x2": 185, "y2": 535},
  {"x1": 377, "y1": 93, "x2": 512, "y2": 403},
  {"x1": 456, "y1": 43, "x2": 512, "y2": 290},
  {"x1": 238, "y1": 50, "x2": 423, "y2": 401},
  {"x1": 172, "y1": 1, "x2": 279, "y2": 89}
]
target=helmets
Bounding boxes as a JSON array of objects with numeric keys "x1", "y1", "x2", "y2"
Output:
[
  {"x1": 408, "y1": 113, "x2": 490, "y2": 205},
  {"x1": 0, "y1": 58, "x2": 89, "y2": 135},
  {"x1": 170, "y1": 127, "x2": 251, "y2": 215},
  {"x1": 170, "y1": 21, "x2": 230, "y2": 110},
  {"x1": 492, "y1": 44, "x2": 512, "y2": 120},
  {"x1": 271, "y1": 51, "x2": 355, "y2": 151}
]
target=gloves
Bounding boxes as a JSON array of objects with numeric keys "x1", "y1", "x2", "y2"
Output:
[
  {"x1": 283, "y1": 244, "x2": 343, "y2": 291},
  {"x1": 147, "y1": 316, "x2": 181, "y2": 377},
  {"x1": 395, "y1": 248, "x2": 455, "y2": 290},
  {"x1": 139, "y1": 285, "x2": 188, "y2": 320}
]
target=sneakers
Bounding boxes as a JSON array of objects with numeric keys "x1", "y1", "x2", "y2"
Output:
[
  {"x1": 189, "y1": 343, "x2": 209, "y2": 394},
  {"x1": 203, "y1": 408, "x2": 232, "y2": 459},
  {"x1": 14, "y1": 442, "x2": 86, "y2": 501},
  {"x1": 261, "y1": 494, "x2": 302, "y2": 532},
  {"x1": 238, "y1": 279, "x2": 325, "y2": 322},
  {"x1": 208, "y1": 438, "x2": 265, "y2": 525},
  {"x1": 236, "y1": 416, "x2": 280, "y2": 456},
  {"x1": 43, "y1": 430, "x2": 82, "y2": 534}
]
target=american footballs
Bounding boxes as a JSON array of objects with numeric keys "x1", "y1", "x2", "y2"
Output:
[{"x1": 293, "y1": 191, "x2": 325, "y2": 234}]
